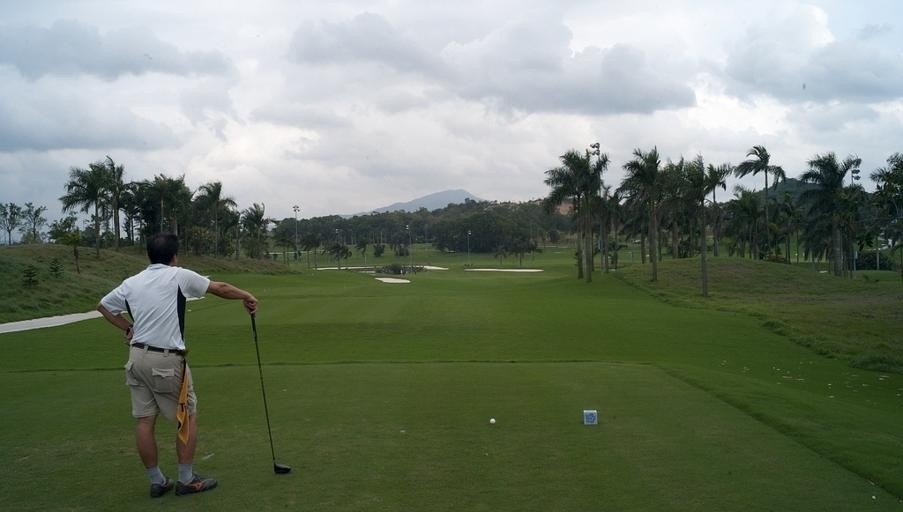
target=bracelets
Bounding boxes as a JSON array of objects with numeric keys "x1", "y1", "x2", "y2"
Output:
[{"x1": 126, "y1": 324, "x2": 133, "y2": 334}]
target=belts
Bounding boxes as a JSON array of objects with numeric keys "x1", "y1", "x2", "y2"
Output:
[{"x1": 131, "y1": 342, "x2": 179, "y2": 353}]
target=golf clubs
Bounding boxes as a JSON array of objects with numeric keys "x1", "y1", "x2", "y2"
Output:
[{"x1": 251, "y1": 315, "x2": 290, "y2": 475}]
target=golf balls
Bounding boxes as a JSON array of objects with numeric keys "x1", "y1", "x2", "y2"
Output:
[{"x1": 490, "y1": 418, "x2": 495, "y2": 424}]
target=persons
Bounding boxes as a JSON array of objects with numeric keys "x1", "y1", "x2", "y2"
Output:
[{"x1": 96, "y1": 231, "x2": 259, "y2": 498}]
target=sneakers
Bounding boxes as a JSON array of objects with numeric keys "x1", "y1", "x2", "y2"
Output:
[
  {"x1": 175, "y1": 473, "x2": 218, "y2": 495},
  {"x1": 150, "y1": 478, "x2": 175, "y2": 497}
]
